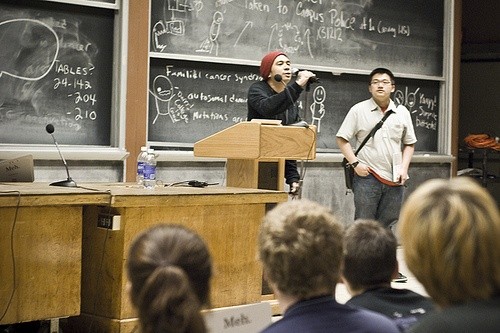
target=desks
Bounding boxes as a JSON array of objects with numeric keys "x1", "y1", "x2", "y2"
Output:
[
  {"x1": 0, "y1": 181, "x2": 112, "y2": 333},
  {"x1": 79, "y1": 182, "x2": 287, "y2": 333}
]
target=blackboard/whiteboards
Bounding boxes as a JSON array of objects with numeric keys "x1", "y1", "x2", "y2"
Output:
[
  {"x1": 149, "y1": 0, "x2": 447, "y2": 80},
  {"x1": 146, "y1": 57, "x2": 441, "y2": 156},
  {"x1": 0, "y1": 0, "x2": 119, "y2": 149}
]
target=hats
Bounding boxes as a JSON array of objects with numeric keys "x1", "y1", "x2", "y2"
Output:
[{"x1": 259, "y1": 51, "x2": 289, "y2": 80}]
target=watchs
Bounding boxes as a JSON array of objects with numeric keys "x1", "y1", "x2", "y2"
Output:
[{"x1": 351, "y1": 160, "x2": 359, "y2": 168}]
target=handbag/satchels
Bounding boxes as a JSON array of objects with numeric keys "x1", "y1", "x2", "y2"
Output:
[{"x1": 341, "y1": 157, "x2": 354, "y2": 188}]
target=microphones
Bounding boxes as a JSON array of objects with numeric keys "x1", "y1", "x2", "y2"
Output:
[
  {"x1": 273, "y1": 74, "x2": 309, "y2": 127},
  {"x1": 291, "y1": 67, "x2": 320, "y2": 83},
  {"x1": 46, "y1": 123, "x2": 76, "y2": 187}
]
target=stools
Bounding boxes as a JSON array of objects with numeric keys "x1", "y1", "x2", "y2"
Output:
[{"x1": 457, "y1": 145, "x2": 498, "y2": 188}]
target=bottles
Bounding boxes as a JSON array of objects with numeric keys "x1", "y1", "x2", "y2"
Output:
[
  {"x1": 136, "y1": 147, "x2": 147, "y2": 186},
  {"x1": 144, "y1": 149, "x2": 156, "y2": 187}
]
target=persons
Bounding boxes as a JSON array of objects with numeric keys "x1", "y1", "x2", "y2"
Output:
[
  {"x1": 125, "y1": 224, "x2": 213, "y2": 333},
  {"x1": 257, "y1": 199, "x2": 399, "y2": 333},
  {"x1": 399, "y1": 177, "x2": 500, "y2": 333},
  {"x1": 340, "y1": 219, "x2": 436, "y2": 333},
  {"x1": 248, "y1": 51, "x2": 315, "y2": 192},
  {"x1": 335, "y1": 68, "x2": 418, "y2": 229}
]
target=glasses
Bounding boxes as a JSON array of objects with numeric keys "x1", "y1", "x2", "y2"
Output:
[{"x1": 368, "y1": 80, "x2": 393, "y2": 85}]
